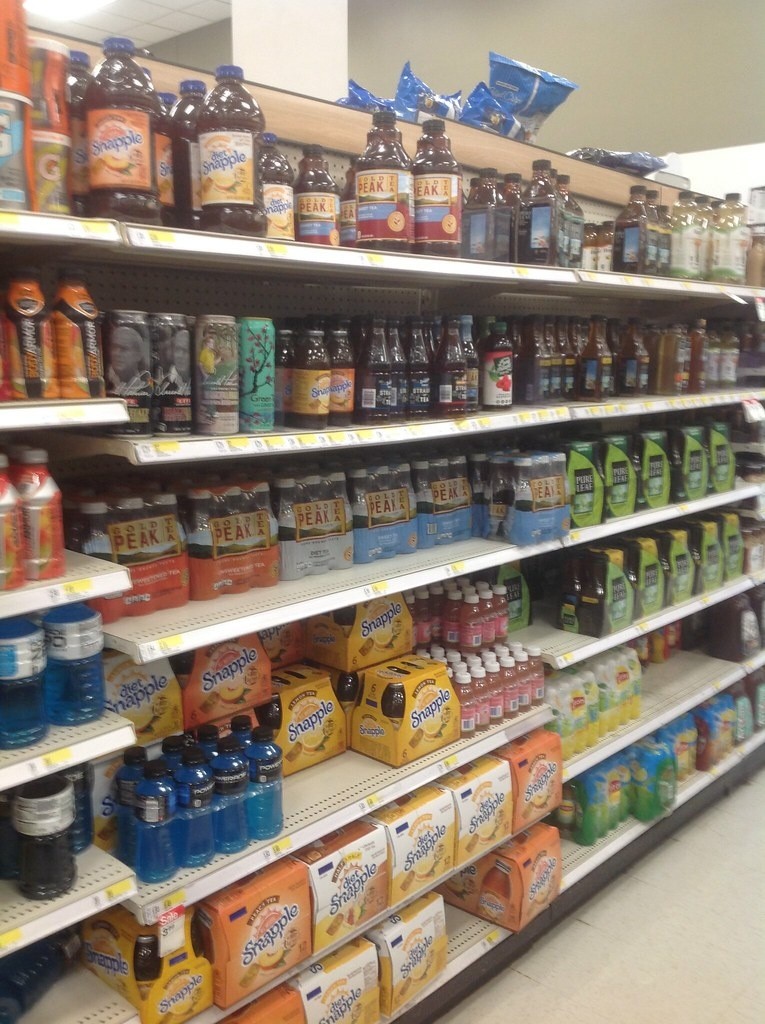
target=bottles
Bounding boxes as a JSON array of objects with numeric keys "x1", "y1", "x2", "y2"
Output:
[
  {"x1": 66, "y1": 414, "x2": 765, "y2": 998},
  {"x1": 0, "y1": 444, "x2": 66, "y2": 590},
  {"x1": 0, "y1": 603, "x2": 106, "y2": 1024},
  {"x1": 52, "y1": 270, "x2": 105, "y2": 399},
  {"x1": 66, "y1": 38, "x2": 749, "y2": 285},
  {"x1": 275, "y1": 316, "x2": 765, "y2": 430},
  {"x1": 5, "y1": 266, "x2": 59, "y2": 400}
]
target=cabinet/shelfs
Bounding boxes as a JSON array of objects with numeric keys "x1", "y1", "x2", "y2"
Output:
[{"x1": 0, "y1": 25, "x2": 764, "y2": 1024}]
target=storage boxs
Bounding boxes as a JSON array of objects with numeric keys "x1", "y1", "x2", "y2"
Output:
[{"x1": 193, "y1": 731, "x2": 563, "y2": 1024}]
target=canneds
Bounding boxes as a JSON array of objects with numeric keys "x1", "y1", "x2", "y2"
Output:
[{"x1": 96, "y1": 308, "x2": 274, "y2": 438}]
